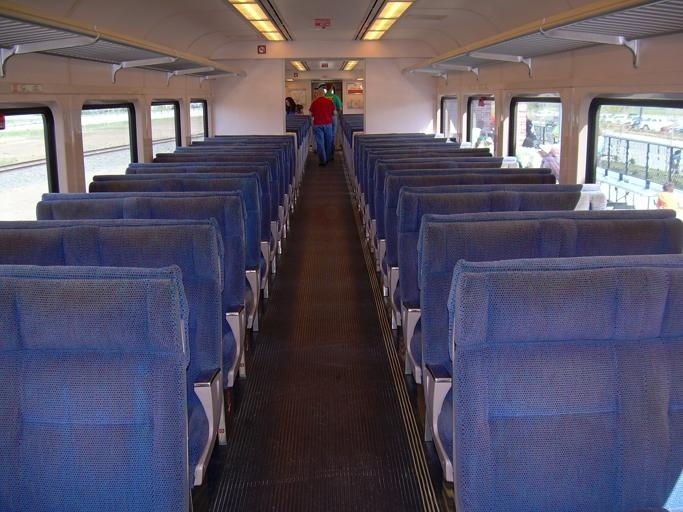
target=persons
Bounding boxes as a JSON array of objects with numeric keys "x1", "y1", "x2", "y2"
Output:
[
  {"x1": 522, "y1": 120, "x2": 539, "y2": 148},
  {"x1": 656, "y1": 183, "x2": 683, "y2": 211},
  {"x1": 286, "y1": 82, "x2": 343, "y2": 167},
  {"x1": 475, "y1": 114, "x2": 495, "y2": 149},
  {"x1": 538, "y1": 143, "x2": 559, "y2": 183}
]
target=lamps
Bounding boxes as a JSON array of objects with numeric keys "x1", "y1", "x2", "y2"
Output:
[
  {"x1": 338, "y1": 60, "x2": 360, "y2": 72},
  {"x1": 222, "y1": 0, "x2": 294, "y2": 44},
  {"x1": 288, "y1": 59, "x2": 311, "y2": 72},
  {"x1": 353, "y1": 1, "x2": 416, "y2": 42}
]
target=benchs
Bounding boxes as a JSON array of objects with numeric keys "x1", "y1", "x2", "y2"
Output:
[
  {"x1": 1, "y1": 219, "x2": 229, "y2": 486},
  {"x1": 340, "y1": 114, "x2": 609, "y2": 444},
  {"x1": 38, "y1": 116, "x2": 311, "y2": 388},
  {"x1": 418, "y1": 208, "x2": 682, "y2": 483},
  {"x1": 446, "y1": 253, "x2": 682, "y2": 510},
  {"x1": 1, "y1": 262, "x2": 192, "y2": 511}
]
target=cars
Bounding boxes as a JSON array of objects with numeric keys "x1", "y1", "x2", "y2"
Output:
[{"x1": 600, "y1": 112, "x2": 675, "y2": 132}]
